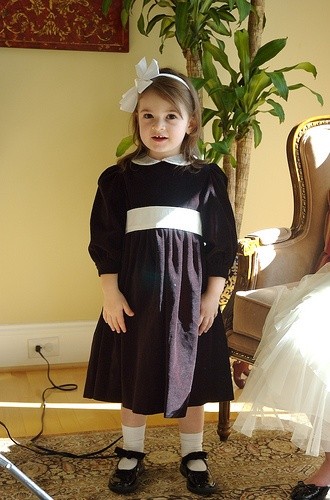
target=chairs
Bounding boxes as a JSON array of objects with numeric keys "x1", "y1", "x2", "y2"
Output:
[{"x1": 217, "y1": 115, "x2": 330, "y2": 442}]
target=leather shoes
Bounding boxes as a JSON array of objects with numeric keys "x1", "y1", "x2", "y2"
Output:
[
  {"x1": 108, "y1": 447, "x2": 146, "y2": 493},
  {"x1": 180, "y1": 451, "x2": 216, "y2": 494}
]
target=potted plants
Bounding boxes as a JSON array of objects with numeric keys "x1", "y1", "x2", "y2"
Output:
[{"x1": 103, "y1": 0, "x2": 325, "y2": 336}]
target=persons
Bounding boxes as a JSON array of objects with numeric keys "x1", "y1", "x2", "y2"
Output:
[
  {"x1": 83, "y1": 55, "x2": 237, "y2": 496},
  {"x1": 230, "y1": 262, "x2": 330, "y2": 500}
]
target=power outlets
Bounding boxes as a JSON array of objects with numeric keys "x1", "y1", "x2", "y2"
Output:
[{"x1": 28, "y1": 336, "x2": 61, "y2": 358}]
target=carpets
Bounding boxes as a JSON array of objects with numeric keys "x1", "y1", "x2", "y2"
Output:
[{"x1": 0, "y1": 419, "x2": 325, "y2": 500}]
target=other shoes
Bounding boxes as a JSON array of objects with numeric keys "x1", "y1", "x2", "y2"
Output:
[{"x1": 290, "y1": 481, "x2": 329, "y2": 500}]
